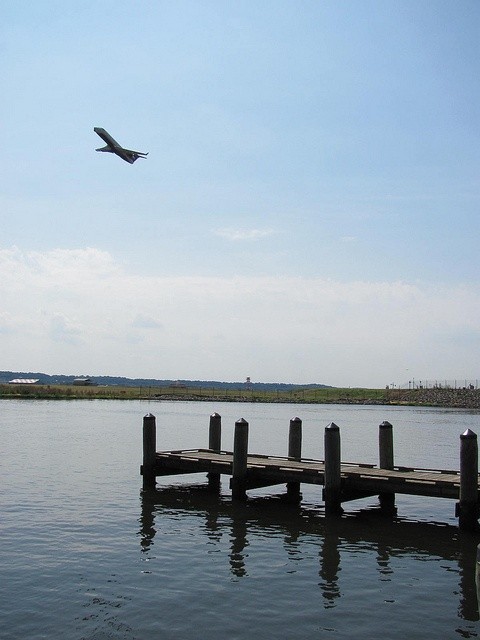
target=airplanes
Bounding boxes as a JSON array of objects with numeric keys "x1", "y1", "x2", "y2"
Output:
[{"x1": 93, "y1": 127, "x2": 149, "y2": 165}]
